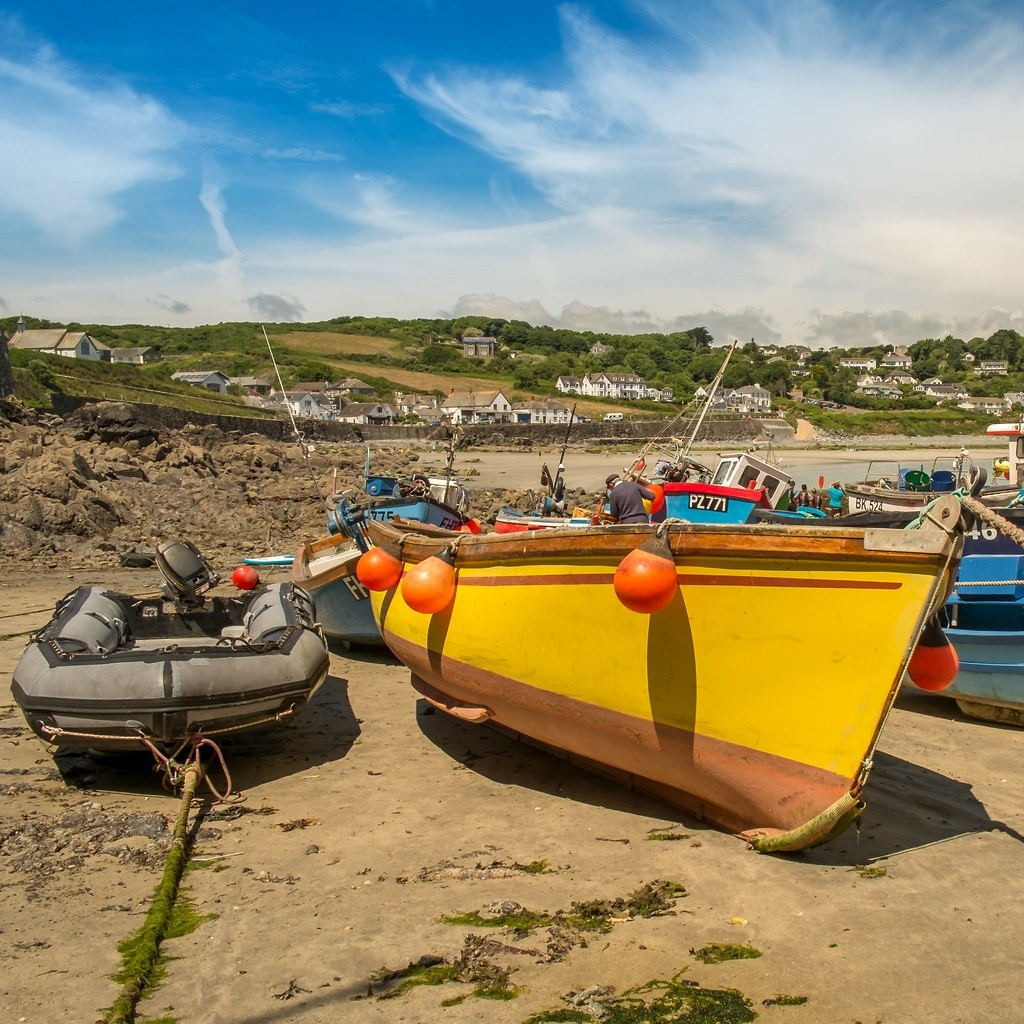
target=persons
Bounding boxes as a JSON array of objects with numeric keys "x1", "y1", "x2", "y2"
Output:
[
  {"x1": 604, "y1": 472, "x2": 657, "y2": 525},
  {"x1": 793, "y1": 483, "x2": 814, "y2": 510},
  {"x1": 810, "y1": 487, "x2": 826, "y2": 513},
  {"x1": 952, "y1": 455, "x2": 961, "y2": 471},
  {"x1": 786, "y1": 478, "x2": 796, "y2": 506},
  {"x1": 827, "y1": 480, "x2": 848, "y2": 518}
]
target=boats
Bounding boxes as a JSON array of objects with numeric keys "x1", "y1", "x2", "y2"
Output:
[
  {"x1": 293, "y1": 531, "x2": 392, "y2": 664},
  {"x1": 327, "y1": 445, "x2": 468, "y2": 537},
  {"x1": 9, "y1": 537, "x2": 332, "y2": 773},
  {"x1": 324, "y1": 465, "x2": 990, "y2": 861},
  {"x1": 492, "y1": 338, "x2": 1024, "y2": 738},
  {"x1": 242, "y1": 554, "x2": 295, "y2": 564}
]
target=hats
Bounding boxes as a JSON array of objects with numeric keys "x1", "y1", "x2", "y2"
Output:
[{"x1": 606, "y1": 474, "x2": 619, "y2": 484}]
